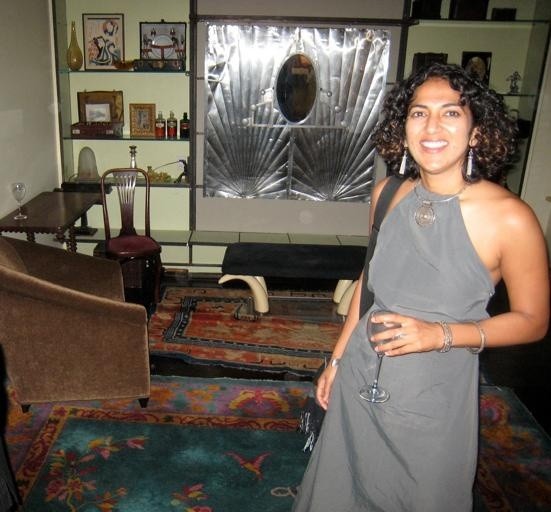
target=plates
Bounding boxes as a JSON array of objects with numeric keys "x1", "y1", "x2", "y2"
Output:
[{"x1": 151, "y1": 36, "x2": 174, "y2": 57}]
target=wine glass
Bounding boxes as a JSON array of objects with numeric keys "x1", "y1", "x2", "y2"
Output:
[
  {"x1": 356, "y1": 309, "x2": 401, "y2": 404},
  {"x1": 10, "y1": 182, "x2": 30, "y2": 221}
]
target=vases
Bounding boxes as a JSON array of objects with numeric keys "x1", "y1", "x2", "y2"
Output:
[{"x1": 66, "y1": 21, "x2": 84, "y2": 71}]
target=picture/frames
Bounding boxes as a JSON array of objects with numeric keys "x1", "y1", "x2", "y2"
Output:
[
  {"x1": 130, "y1": 104, "x2": 157, "y2": 137},
  {"x1": 462, "y1": 51, "x2": 493, "y2": 90},
  {"x1": 139, "y1": 21, "x2": 187, "y2": 71},
  {"x1": 83, "y1": 12, "x2": 126, "y2": 71}
]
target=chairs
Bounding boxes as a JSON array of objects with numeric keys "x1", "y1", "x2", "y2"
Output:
[{"x1": 100, "y1": 167, "x2": 165, "y2": 304}]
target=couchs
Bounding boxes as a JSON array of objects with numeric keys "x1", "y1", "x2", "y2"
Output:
[{"x1": 0, "y1": 233, "x2": 151, "y2": 414}]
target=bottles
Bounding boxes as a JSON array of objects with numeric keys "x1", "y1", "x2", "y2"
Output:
[
  {"x1": 155, "y1": 111, "x2": 165, "y2": 138},
  {"x1": 166, "y1": 110, "x2": 177, "y2": 138},
  {"x1": 179, "y1": 111, "x2": 190, "y2": 138}
]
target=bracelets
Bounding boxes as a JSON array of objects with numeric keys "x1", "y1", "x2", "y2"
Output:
[
  {"x1": 433, "y1": 320, "x2": 454, "y2": 354},
  {"x1": 327, "y1": 357, "x2": 342, "y2": 368},
  {"x1": 461, "y1": 320, "x2": 487, "y2": 354}
]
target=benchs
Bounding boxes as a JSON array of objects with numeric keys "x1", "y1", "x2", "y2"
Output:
[{"x1": 218, "y1": 242, "x2": 368, "y2": 316}]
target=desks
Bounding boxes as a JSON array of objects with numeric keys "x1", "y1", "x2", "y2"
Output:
[{"x1": 0, "y1": 190, "x2": 112, "y2": 252}]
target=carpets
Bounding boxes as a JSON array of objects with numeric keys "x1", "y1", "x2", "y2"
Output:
[
  {"x1": 0, "y1": 376, "x2": 551, "y2": 512},
  {"x1": 148, "y1": 285, "x2": 347, "y2": 377}
]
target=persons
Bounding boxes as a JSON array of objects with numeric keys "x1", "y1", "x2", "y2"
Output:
[
  {"x1": 289, "y1": 60, "x2": 551, "y2": 511},
  {"x1": 92, "y1": 22, "x2": 118, "y2": 66}
]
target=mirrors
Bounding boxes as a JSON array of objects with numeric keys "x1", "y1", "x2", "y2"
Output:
[{"x1": 276, "y1": 52, "x2": 322, "y2": 125}]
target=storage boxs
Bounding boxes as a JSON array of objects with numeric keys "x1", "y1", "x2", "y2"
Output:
[{"x1": 69, "y1": 88, "x2": 126, "y2": 140}]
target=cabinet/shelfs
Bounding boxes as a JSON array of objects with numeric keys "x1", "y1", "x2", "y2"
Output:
[
  {"x1": 398, "y1": 0, "x2": 551, "y2": 199},
  {"x1": 52, "y1": 1, "x2": 198, "y2": 232}
]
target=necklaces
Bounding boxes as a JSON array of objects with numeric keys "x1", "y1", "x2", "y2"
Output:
[{"x1": 412, "y1": 173, "x2": 473, "y2": 230}]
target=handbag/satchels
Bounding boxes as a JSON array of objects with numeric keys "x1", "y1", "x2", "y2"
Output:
[{"x1": 295, "y1": 397, "x2": 325, "y2": 463}]
